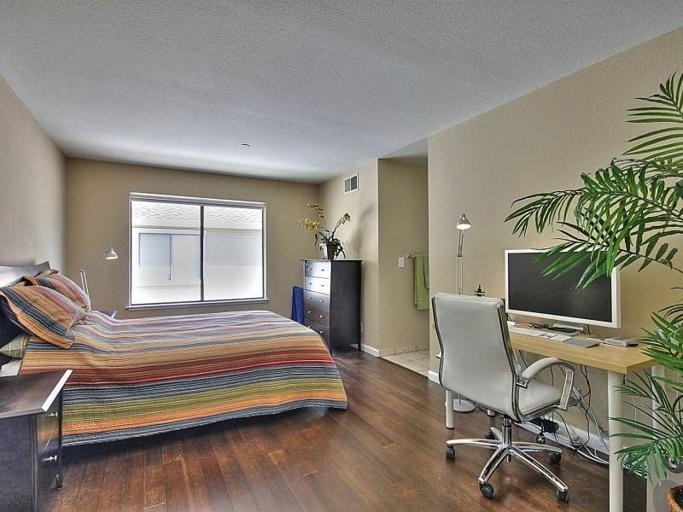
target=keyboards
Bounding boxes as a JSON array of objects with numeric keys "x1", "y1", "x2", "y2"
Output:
[{"x1": 508, "y1": 326, "x2": 573, "y2": 343}]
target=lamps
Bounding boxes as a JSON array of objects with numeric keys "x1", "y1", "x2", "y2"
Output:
[
  {"x1": 455, "y1": 210, "x2": 472, "y2": 295},
  {"x1": 78, "y1": 245, "x2": 119, "y2": 309}
]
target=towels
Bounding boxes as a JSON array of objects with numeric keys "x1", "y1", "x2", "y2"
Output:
[{"x1": 413, "y1": 256, "x2": 429, "y2": 310}]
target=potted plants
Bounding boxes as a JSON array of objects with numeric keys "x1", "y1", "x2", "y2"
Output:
[{"x1": 507, "y1": 125, "x2": 683, "y2": 512}]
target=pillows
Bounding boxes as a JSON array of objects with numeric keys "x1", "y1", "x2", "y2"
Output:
[{"x1": 0, "y1": 269, "x2": 93, "y2": 361}]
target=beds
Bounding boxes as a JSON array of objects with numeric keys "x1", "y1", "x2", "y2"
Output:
[{"x1": 0, "y1": 261, "x2": 347, "y2": 448}]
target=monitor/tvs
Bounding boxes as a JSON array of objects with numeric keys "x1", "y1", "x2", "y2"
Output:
[{"x1": 505, "y1": 250, "x2": 622, "y2": 336}]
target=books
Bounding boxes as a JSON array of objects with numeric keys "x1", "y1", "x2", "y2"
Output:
[{"x1": 604, "y1": 335, "x2": 642, "y2": 347}]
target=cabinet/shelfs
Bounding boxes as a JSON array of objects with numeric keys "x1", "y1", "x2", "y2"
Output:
[{"x1": 300, "y1": 256, "x2": 364, "y2": 356}]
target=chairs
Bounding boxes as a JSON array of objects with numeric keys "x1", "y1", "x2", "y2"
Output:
[{"x1": 429, "y1": 292, "x2": 580, "y2": 503}]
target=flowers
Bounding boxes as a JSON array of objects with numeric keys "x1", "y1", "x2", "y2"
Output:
[{"x1": 300, "y1": 199, "x2": 353, "y2": 258}]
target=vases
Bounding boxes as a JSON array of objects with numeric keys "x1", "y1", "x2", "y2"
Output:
[{"x1": 326, "y1": 241, "x2": 335, "y2": 261}]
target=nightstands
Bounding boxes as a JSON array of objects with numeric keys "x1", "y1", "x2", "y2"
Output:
[{"x1": 1, "y1": 369, "x2": 74, "y2": 512}]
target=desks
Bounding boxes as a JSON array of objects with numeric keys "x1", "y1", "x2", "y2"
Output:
[{"x1": 496, "y1": 309, "x2": 672, "y2": 512}]
